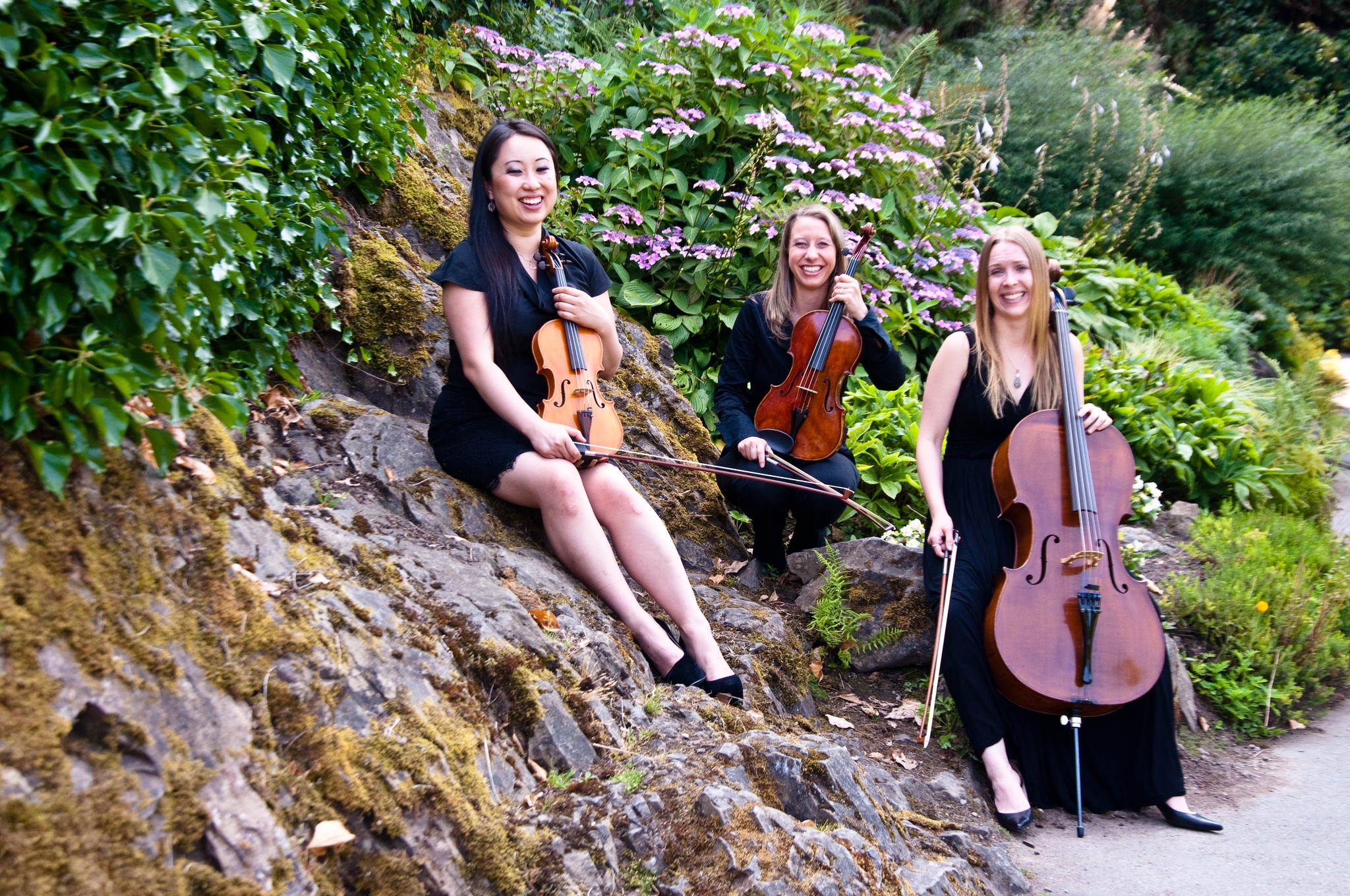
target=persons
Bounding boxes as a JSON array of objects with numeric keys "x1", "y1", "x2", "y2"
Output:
[
  {"x1": 429, "y1": 115, "x2": 746, "y2": 711},
  {"x1": 917, "y1": 224, "x2": 1228, "y2": 835},
  {"x1": 707, "y1": 203, "x2": 907, "y2": 571}
]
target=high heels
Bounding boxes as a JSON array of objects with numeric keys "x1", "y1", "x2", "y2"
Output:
[
  {"x1": 677, "y1": 631, "x2": 743, "y2": 702},
  {"x1": 633, "y1": 617, "x2": 708, "y2": 689},
  {"x1": 1136, "y1": 801, "x2": 1224, "y2": 832},
  {"x1": 994, "y1": 763, "x2": 1033, "y2": 831}
]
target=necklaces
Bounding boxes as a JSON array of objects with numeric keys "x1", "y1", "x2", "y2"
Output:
[
  {"x1": 513, "y1": 247, "x2": 540, "y2": 271},
  {"x1": 790, "y1": 304, "x2": 801, "y2": 321},
  {"x1": 995, "y1": 327, "x2": 1032, "y2": 389}
]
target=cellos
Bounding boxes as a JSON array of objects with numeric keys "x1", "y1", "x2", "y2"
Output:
[{"x1": 986, "y1": 257, "x2": 1168, "y2": 840}]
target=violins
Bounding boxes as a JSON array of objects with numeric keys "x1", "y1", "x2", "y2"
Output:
[
  {"x1": 752, "y1": 222, "x2": 875, "y2": 463},
  {"x1": 530, "y1": 235, "x2": 624, "y2": 469}
]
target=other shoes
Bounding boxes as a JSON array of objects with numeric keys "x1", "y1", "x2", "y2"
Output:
[{"x1": 751, "y1": 527, "x2": 829, "y2": 567}]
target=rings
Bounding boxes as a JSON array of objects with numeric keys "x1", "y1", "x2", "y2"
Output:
[{"x1": 931, "y1": 544, "x2": 941, "y2": 549}]
